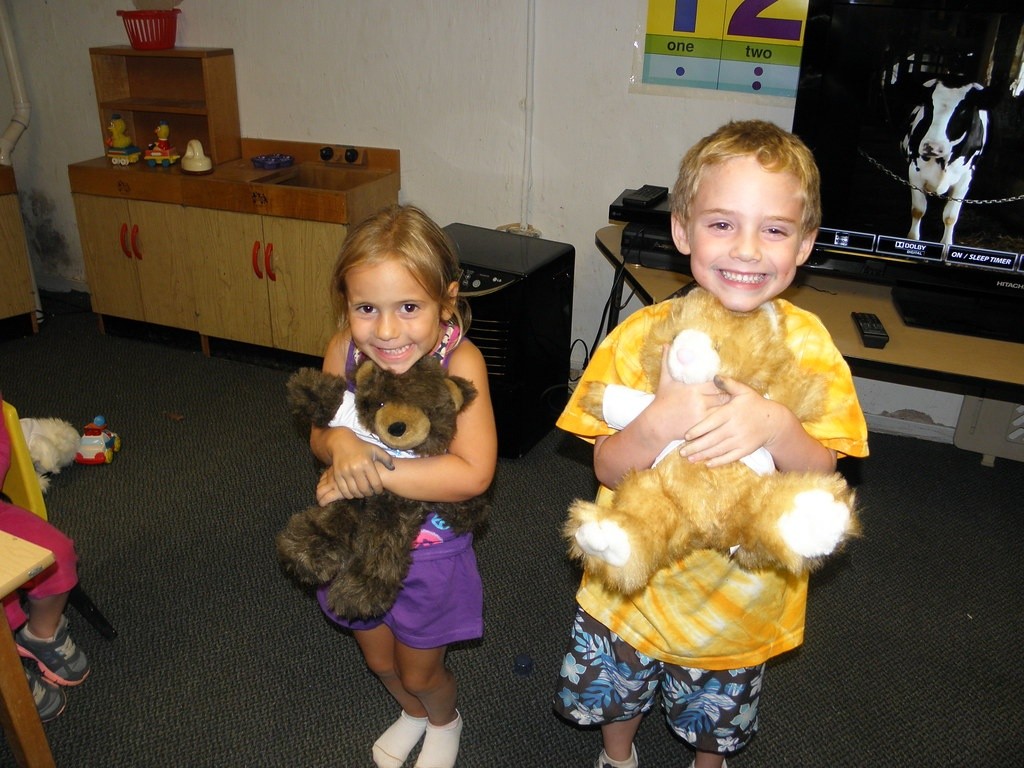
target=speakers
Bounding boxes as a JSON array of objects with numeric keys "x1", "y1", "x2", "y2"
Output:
[{"x1": 441, "y1": 222, "x2": 575, "y2": 460}]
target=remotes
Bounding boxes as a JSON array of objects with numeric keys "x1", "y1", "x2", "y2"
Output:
[
  {"x1": 852, "y1": 313, "x2": 890, "y2": 349},
  {"x1": 623, "y1": 184, "x2": 669, "y2": 207}
]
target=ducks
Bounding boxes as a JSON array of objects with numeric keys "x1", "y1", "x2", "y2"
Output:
[
  {"x1": 155, "y1": 121, "x2": 170, "y2": 150},
  {"x1": 106, "y1": 113, "x2": 132, "y2": 150}
]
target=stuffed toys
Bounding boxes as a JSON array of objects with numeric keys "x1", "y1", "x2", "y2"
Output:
[
  {"x1": 276, "y1": 355, "x2": 493, "y2": 626},
  {"x1": 556, "y1": 283, "x2": 868, "y2": 599},
  {"x1": 18, "y1": 417, "x2": 81, "y2": 492}
]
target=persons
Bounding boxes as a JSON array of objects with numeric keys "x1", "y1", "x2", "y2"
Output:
[
  {"x1": 554, "y1": 118, "x2": 869, "y2": 768},
  {"x1": 0, "y1": 391, "x2": 93, "y2": 722},
  {"x1": 311, "y1": 210, "x2": 498, "y2": 768}
]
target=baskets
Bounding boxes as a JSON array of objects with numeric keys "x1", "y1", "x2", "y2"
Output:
[{"x1": 116, "y1": 8, "x2": 182, "y2": 50}]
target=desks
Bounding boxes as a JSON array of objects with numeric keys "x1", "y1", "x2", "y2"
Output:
[
  {"x1": 0, "y1": 529, "x2": 56, "y2": 768},
  {"x1": 593, "y1": 225, "x2": 1024, "y2": 406}
]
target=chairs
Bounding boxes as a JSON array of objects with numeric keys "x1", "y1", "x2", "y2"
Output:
[{"x1": 2, "y1": 399, "x2": 119, "y2": 642}]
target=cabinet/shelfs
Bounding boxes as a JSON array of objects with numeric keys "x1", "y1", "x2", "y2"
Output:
[
  {"x1": 185, "y1": 192, "x2": 399, "y2": 360},
  {"x1": 72, "y1": 192, "x2": 195, "y2": 334},
  {"x1": 88, "y1": 45, "x2": 243, "y2": 165}
]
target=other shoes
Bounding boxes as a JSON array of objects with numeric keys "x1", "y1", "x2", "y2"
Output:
[{"x1": 590, "y1": 742, "x2": 639, "y2": 768}]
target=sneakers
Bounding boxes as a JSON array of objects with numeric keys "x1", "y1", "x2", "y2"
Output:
[
  {"x1": 22, "y1": 663, "x2": 68, "y2": 723},
  {"x1": 15, "y1": 615, "x2": 91, "y2": 687}
]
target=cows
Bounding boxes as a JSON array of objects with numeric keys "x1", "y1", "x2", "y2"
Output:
[{"x1": 897, "y1": 65, "x2": 993, "y2": 262}]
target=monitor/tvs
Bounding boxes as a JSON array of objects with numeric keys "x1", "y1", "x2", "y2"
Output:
[{"x1": 790, "y1": 0, "x2": 1024, "y2": 344}]
